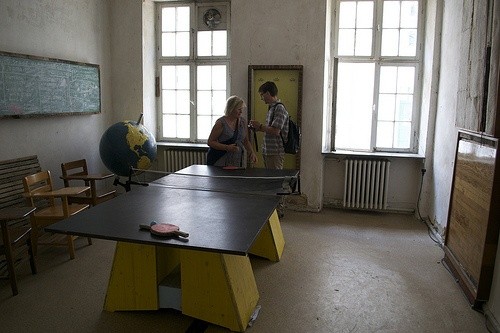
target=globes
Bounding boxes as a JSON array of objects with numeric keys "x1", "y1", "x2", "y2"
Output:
[{"x1": 99, "y1": 113, "x2": 158, "y2": 192}]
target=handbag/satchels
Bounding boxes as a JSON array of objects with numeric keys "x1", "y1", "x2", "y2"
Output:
[{"x1": 206, "y1": 122, "x2": 239, "y2": 165}]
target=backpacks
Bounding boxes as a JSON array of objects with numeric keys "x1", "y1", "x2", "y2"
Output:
[{"x1": 268, "y1": 102, "x2": 300, "y2": 155}]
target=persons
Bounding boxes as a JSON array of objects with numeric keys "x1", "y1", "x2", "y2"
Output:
[
  {"x1": 207, "y1": 95, "x2": 257, "y2": 168},
  {"x1": 249, "y1": 81, "x2": 289, "y2": 219}
]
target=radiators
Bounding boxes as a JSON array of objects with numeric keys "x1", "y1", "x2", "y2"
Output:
[
  {"x1": 342, "y1": 159, "x2": 391, "y2": 210},
  {"x1": 159, "y1": 146, "x2": 209, "y2": 172}
]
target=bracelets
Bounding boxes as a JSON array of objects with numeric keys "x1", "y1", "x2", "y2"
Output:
[{"x1": 260, "y1": 124, "x2": 263, "y2": 129}]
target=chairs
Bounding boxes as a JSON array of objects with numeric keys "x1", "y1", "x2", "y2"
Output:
[
  {"x1": 0, "y1": 207, "x2": 38, "y2": 296},
  {"x1": 60, "y1": 158, "x2": 117, "y2": 207},
  {"x1": 21, "y1": 170, "x2": 92, "y2": 260}
]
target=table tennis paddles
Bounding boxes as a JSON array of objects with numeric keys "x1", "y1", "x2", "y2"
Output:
[
  {"x1": 139, "y1": 223, "x2": 172, "y2": 235},
  {"x1": 150, "y1": 223, "x2": 189, "y2": 239}
]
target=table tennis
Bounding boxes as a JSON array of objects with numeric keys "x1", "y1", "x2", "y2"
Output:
[{"x1": 151, "y1": 220, "x2": 157, "y2": 225}]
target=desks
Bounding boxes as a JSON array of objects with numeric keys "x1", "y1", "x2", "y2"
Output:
[
  {"x1": 60, "y1": 172, "x2": 116, "y2": 197},
  {"x1": 45, "y1": 164, "x2": 300, "y2": 333},
  {"x1": 23, "y1": 186, "x2": 90, "y2": 216}
]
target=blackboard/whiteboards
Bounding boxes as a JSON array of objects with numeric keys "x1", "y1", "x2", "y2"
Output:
[{"x1": 0, "y1": 51, "x2": 102, "y2": 119}]
